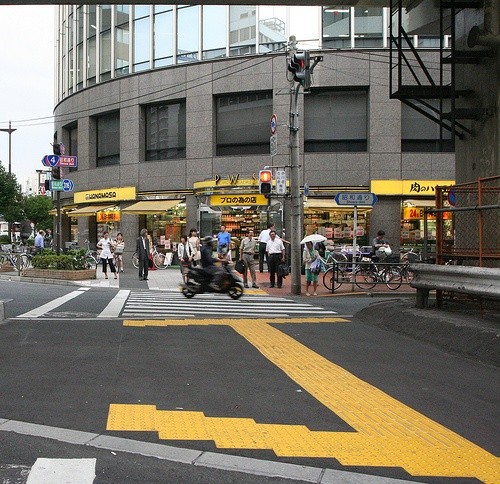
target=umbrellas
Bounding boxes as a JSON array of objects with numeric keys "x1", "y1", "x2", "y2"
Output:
[{"x1": 300, "y1": 234, "x2": 327, "y2": 258}]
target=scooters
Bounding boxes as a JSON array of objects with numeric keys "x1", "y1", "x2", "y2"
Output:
[{"x1": 179, "y1": 248, "x2": 246, "y2": 299}]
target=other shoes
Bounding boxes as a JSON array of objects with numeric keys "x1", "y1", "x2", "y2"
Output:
[
  {"x1": 252, "y1": 285, "x2": 259, "y2": 288},
  {"x1": 269, "y1": 286, "x2": 274, "y2": 288},
  {"x1": 144, "y1": 277, "x2": 148, "y2": 280},
  {"x1": 278, "y1": 285, "x2": 281, "y2": 288},
  {"x1": 244, "y1": 285, "x2": 249, "y2": 288},
  {"x1": 139, "y1": 277, "x2": 143, "y2": 281}
]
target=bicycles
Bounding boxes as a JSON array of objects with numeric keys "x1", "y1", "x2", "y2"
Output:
[
  {"x1": 0, "y1": 241, "x2": 32, "y2": 271},
  {"x1": 322, "y1": 248, "x2": 415, "y2": 290},
  {"x1": 131, "y1": 244, "x2": 169, "y2": 269},
  {"x1": 68, "y1": 239, "x2": 102, "y2": 272}
]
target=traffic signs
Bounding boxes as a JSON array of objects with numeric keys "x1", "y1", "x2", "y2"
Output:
[
  {"x1": 40, "y1": 155, "x2": 78, "y2": 168},
  {"x1": 334, "y1": 193, "x2": 378, "y2": 206}
]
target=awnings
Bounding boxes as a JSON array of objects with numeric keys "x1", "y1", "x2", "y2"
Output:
[
  {"x1": 121, "y1": 200, "x2": 183, "y2": 215},
  {"x1": 66, "y1": 205, "x2": 114, "y2": 217},
  {"x1": 47, "y1": 205, "x2": 78, "y2": 215}
]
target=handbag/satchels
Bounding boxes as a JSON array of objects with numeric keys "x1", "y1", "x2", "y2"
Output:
[
  {"x1": 310, "y1": 258, "x2": 321, "y2": 275},
  {"x1": 235, "y1": 260, "x2": 246, "y2": 274},
  {"x1": 194, "y1": 251, "x2": 201, "y2": 261},
  {"x1": 277, "y1": 260, "x2": 290, "y2": 279}
]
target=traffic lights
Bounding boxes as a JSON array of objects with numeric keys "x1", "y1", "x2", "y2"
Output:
[
  {"x1": 293, "y1": 69, "x2": 310, "y2": 88},
  {"x1": 287, "y1": 51, "x2": 310, "y2": 73},
  {"x1": 259, "y1": 169, "x2": 272, "y2": 195}
]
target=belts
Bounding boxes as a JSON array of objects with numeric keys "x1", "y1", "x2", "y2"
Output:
[{"x1": 243, "y1": 252, "x2": 252, "y2": 255}]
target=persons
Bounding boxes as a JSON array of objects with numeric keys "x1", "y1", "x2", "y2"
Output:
[
  {"x1": 187, "y1": 228, "x2": 202, "y2": 266},
  {"x1": 34, "y1": 230, "x2": 44, "y2": 254},
  {"x1": 217, "y1": 224, "x2": 232, "y2": 266},
  {"x1": 235, "y1": 230, "x2": 259, "y2": 288},
  {"x1": 303, "y1": 241, "x2": 320, "y2": 297},
  {"x1": 258, "y1": 222, "x2": 290, "y2": 272},
  {"x1": 200, "y1": 236, "x2": 227, "y2": 291},
  {"x1": 96, "y1": 231, "x2": 117, "y2": 279},
  {"x1": 44, "y1": 228, "x2": 53, "y2": 248},
  {"x1": 368, "y1": 230, "x2": 393, "y2": 272},
  {"x1": 266, "y1": 231, "x2": 285, "y2": 288},
  {"x1": 111, "y1": 233, "x2": 125, "y2": 273},
  {"x1": 178, "y1": 235, "x2": 194, "y2": 283},
  {"x1": 136, "y1": 229, "x2": 154, "y2": 281}
]
